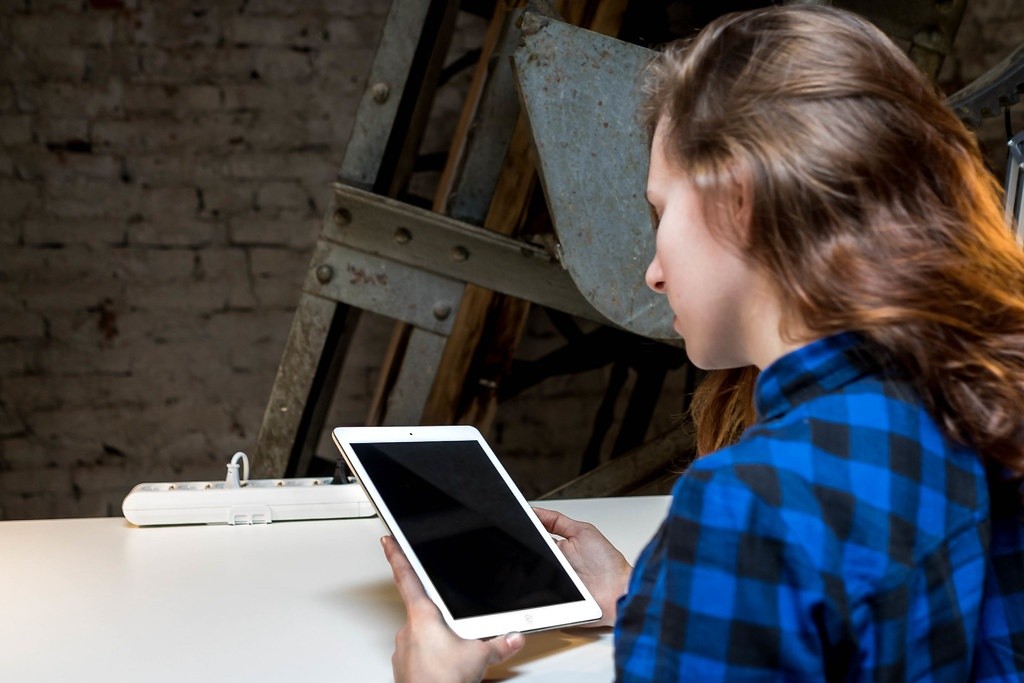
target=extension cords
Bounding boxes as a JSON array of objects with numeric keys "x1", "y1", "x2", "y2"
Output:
[{"x1": 123, "y1": 476, "x2": 376, "y2": 526}]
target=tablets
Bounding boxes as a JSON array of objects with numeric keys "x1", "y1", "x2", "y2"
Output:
[{"x1": 330, "y1": 424, "x2": 602, "y2": 641}]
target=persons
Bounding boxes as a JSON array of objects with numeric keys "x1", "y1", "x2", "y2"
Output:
[{"x1": 379, "y1": 0, "x2": 1024, "y2": 683}]
